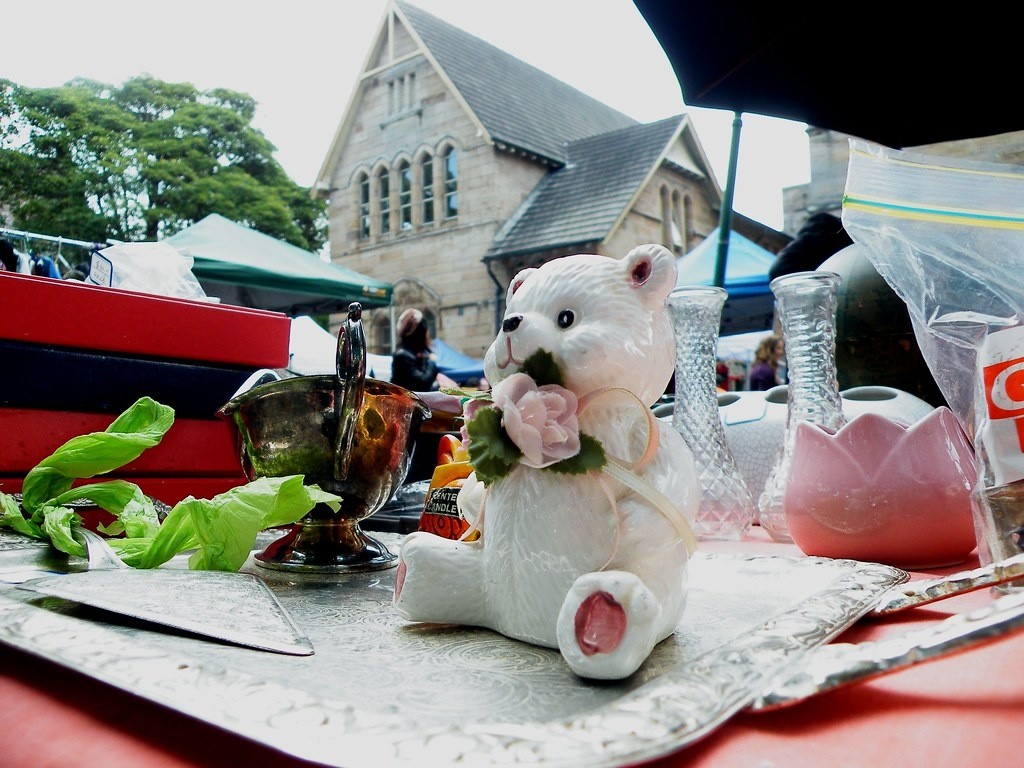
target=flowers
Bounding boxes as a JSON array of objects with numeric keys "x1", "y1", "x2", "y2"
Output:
[{"x1": 457, "y1": 348, "x2": 609, "y2": 489}]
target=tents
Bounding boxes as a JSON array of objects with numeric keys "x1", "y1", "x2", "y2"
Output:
[
  {"x1": 167, "y1": 213, "x2": 397, "y2": 355},
  {"x1": 672, "y1": 222, "x2": 777, "y2": 334},
  {"x1": 428, "y1": 337, "x2": 485, "y2": 386}
]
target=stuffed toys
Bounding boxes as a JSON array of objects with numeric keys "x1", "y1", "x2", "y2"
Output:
[{"x1": 392, "y1": 244, "x2": 701, "y2": 681}]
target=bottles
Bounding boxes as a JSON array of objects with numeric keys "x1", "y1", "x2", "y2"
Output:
[
  {"x1": 663, "y1": 286, "x2": 754, "y2": 540},
  {"x1": 759, "y1": 272, "x2": 848, "y2": 542}
]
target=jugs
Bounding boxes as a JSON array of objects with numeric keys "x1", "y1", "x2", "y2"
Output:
[{"x1": 222, "y1": 303, "x2": 430, "y2": 573}]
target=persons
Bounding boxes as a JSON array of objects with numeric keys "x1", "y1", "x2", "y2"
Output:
[
  {"x1": 386, "y1": 308, "x2": 442, "y2": 484},
  {"x1": 749, "y1": 334, "x2": 785, "y2": 392}
]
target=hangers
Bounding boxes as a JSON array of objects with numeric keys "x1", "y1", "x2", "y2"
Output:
[{"x1": 4, "y1": 228, "x2": 104, "y2": 270}]
